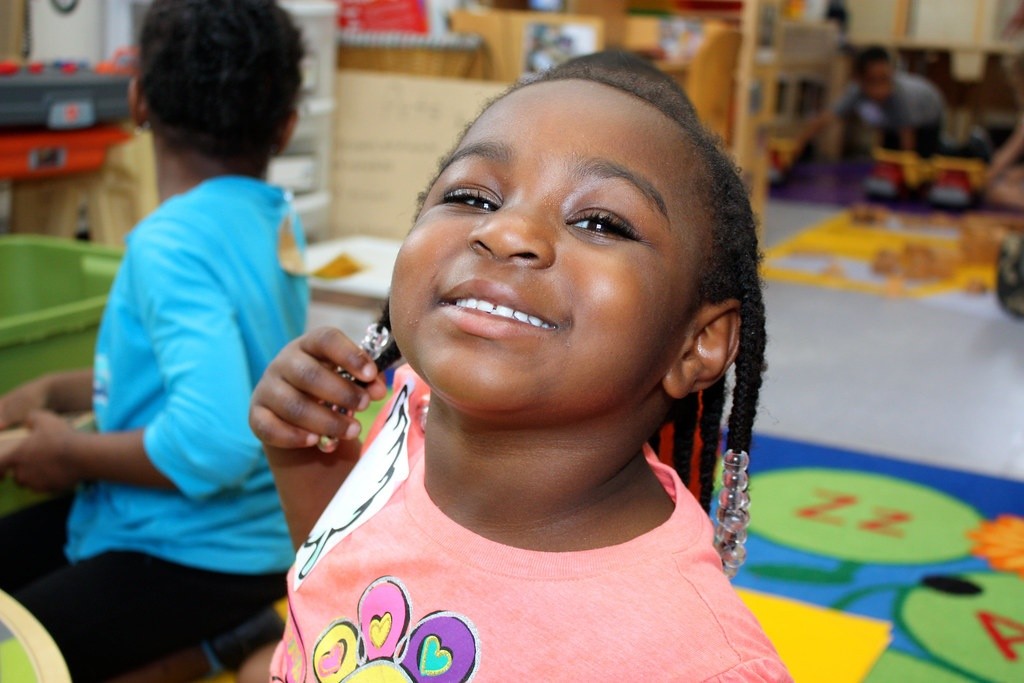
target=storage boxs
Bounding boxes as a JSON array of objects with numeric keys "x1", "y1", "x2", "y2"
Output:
[{"x1": 0, "y1": 233, "x2": 126, "y2": 517}]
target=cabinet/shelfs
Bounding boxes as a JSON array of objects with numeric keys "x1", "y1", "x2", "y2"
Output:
[{"x1": 267, "y1": 4, "x2": 339, "y2": 236}]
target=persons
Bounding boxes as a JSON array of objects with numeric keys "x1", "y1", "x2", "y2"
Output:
[
  {"x1": 1, "y1": 0, "x2": 309, "y2": 683},
  {"x1": 772, "y1": 43, "x2": 994, "y2": 182},
  {"x1": 249, "y1": 46, "x2": 790, "y2": 681}
]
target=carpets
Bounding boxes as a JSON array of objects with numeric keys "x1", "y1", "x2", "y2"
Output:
[
  {"x1": 758, "y1": 210, "x2": 1024, "y2": 305},
  {"x1": 708, "y1": 427, "x2": 1024, "y2": 683}
]
